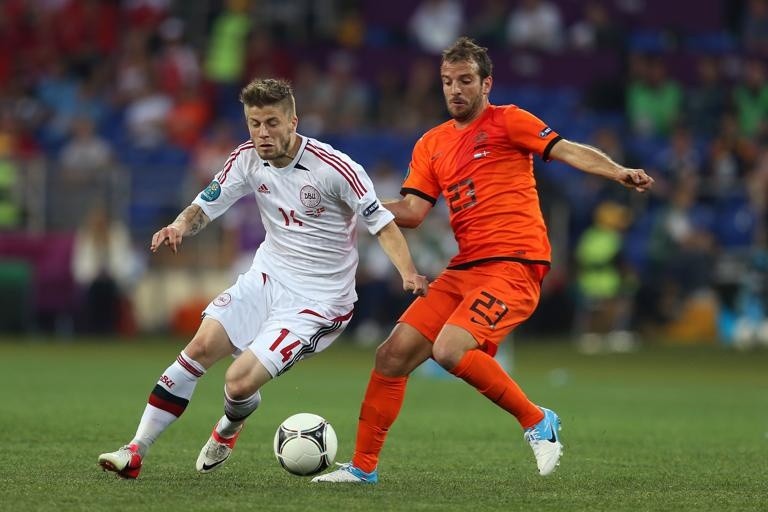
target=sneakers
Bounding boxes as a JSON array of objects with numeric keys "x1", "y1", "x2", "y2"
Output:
[
  {"x1": 99, "y1": 446, "x2": 142, "y2": 478},
  {"x1": 196, "y1": 417, "x2": 242, "y2": 473},
  {"x1": 524, "y1": 405, "x2": 563, "y2": 475},
  {"x1": 311, "y1": 462, "x2": 377, "y2": 483}
]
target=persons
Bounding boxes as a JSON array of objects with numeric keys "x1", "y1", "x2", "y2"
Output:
[
  {"x1": 303, "y1": 34, "x2": 656, "y2": 486},
  {"x1": 1, "y1": 1, "x2": 767, "y2": 384},
  {"x1": 96, "y1": 75, "x2": 432, "y2": 479}
]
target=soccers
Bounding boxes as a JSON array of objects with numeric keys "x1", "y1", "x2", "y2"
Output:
[{"x1": 274, "y1": 413, "x2": 337, "y2": 474}]
